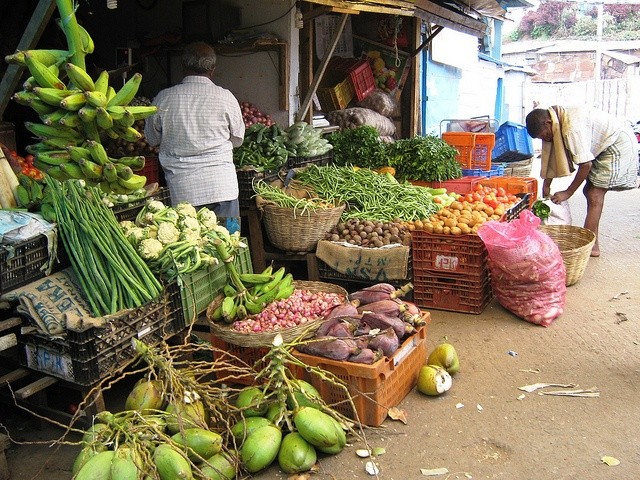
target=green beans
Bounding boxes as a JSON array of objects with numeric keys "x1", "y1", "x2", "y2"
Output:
[{"x1": 251, "y1": 162, "x2": 436, "y2": 219}]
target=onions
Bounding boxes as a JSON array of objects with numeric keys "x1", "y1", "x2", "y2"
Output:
[{"x1": 238, "y1": 102, "x2": 276, "y2": 128}]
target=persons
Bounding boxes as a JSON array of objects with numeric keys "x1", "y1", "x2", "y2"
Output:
[
  {"x1": 526, "y1": 103, "x2": 639, "y2": 257},
  {"x1": 144, "y1": 43, "x2": 246, "y2": 217}
]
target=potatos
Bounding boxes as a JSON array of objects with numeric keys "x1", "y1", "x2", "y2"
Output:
[
  {"x1": 325, "y1": 219, "x2": 409, "y2": 248},
  {"x1": 327, "y1": 90, "x2": 395, "y2": 143},
  {"x1": 410, "y1": 202, "x2": 503, "y2": 235}
]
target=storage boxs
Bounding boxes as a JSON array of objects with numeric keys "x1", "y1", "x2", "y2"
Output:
[
  {"x1": 237, "y1": 171, "x2": 277, "y2": 212},
  {"x1": 461, "y1": 165, "x2": 505, "y2": 180},
  {"x1": 442, "y1": 132, "x2": 496, "y2": 170},
  {"x1": 292, "y1": 310, "x2": 432, "y2": 427},
  {"x1": 314, "y1": 76, "x2": 356, "y2": 112},
  {"x1": 12, "y1": 281, "x2": 186, "y2": 388},
  {"x1": 478, "y1": 176, "x2": 538, "y2": 208},
  {"x1": 410, "y1": 179, "x2": 431, "y2": 186},
  {"x1": 410, "y1": 229, "x2": 489, "y2": 276},
  {"x1": 134, "y1": 155, "x2": 159, "y2": 189},
  {"x1": 210, "y1": 311, "x2": 286, "y2": 389},
  {"x1": 472, "y1": 120, "x2": 534, "y2": 162},
  {"x1": 0, "y1": 227, "x2": 71, "y2": 297},
  {"x1": 112, "y1": 188, "x2": 172, "y2": 224},
  {"x1": 315, "y1": 254, "x2": 414, "y2": 282},
  {"x1": 440, "y1": 176, "x2": 487, "y2": 196},
  {"x1": 462, "y1": 169, "x2": 500, "y2": 179},
  {"x1": 287, "y1": 149, "x2": 334, "y2": 169},
  {"x1": 335, "y1": 56, "x2": 377, "y2": 105},
  {"x1": 413, "y1": 272, "x2": 495, "y2": 315},
  {"x1": 180, "y1": 236, "x2": 253, "y2": 327}
]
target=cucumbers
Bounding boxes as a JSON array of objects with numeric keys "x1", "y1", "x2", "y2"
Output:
[{"x1": 244, "y1": 124, "x2": 285, "y2": 164}]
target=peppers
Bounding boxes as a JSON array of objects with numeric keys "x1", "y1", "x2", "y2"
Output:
[{"x1": 232, "y1": 144, "x2": 268, "y2": 169}]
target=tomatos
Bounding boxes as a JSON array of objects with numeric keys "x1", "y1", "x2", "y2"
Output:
[
  {"x1": 3, "y1": 146, "x2": 44, "y2": 183},
  {"x1": 457, "y1": 184, "x2": 517, "y2": 208}
]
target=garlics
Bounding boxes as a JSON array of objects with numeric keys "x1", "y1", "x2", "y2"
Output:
[{"x1": 234, "y1": 288, "x2": 339, "y2": 333}]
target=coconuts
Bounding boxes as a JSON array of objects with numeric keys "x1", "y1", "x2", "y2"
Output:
[
  {"x1": 418, "y1": 366, "x2": 452, "y2": 396},
  {"x1": 427, "y1": 344, "x2": 458, "y2": 374}
]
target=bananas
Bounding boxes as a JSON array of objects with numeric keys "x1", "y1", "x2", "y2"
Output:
[
  {"x1": 6, "y1": 17, "x2": 156, "y2": 192},
  {"x1": 211, "y1": 261, "x2": 295, "y2": 323}
]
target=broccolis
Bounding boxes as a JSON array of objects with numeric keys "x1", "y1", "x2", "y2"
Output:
[{"x1": 122, "y1": 202, "x2": 245, "y2": 277}]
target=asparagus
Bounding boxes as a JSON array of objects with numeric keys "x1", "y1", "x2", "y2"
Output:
[{"x1": 44, "y1": 173, "x2": 161, "y2": 317}]
